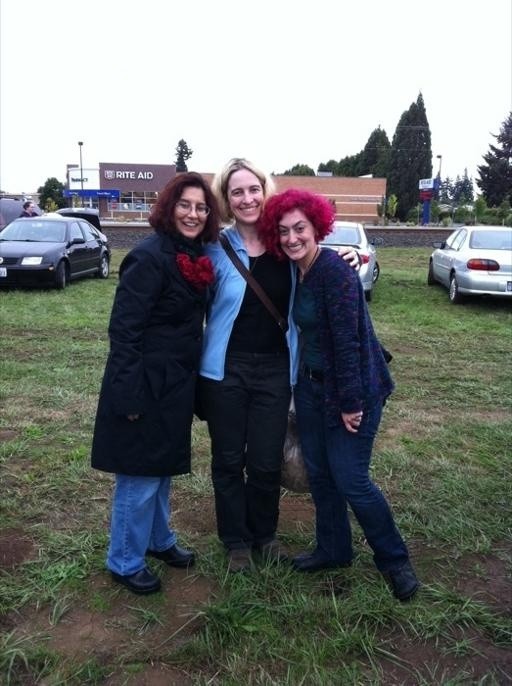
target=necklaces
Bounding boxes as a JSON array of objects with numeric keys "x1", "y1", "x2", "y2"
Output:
[{"x1": 298, "y1": 246, "x2": 320, "y2": 280}]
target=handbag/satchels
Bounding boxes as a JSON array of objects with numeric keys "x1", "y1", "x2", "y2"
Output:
[{"x1": 279, "y1": 410, "x2": 311, "y2": 493}]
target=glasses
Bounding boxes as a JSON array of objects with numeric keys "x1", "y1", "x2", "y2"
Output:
[{"x1": 176, "y1": 201, "x2": 210, "y2": 217}]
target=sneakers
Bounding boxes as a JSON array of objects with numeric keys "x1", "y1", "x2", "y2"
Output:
[
  {"x1": 228, "y1": 548, "x2": 251, "y2": 572},
  {"x1": 258, "y1": 539, "x2": 288, "y2": 561}
]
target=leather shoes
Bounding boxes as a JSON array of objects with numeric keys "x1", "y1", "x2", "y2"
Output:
[
  {"x1": 290, "y1": 548, "x2": 352, "y2": 572},
  {"x1": 111, "y1": 567, "x2": 161, "y2": 593},
  {"x1": 387, "y1": 559, "x2": 418, "y2": 599},
  {"x1": 145, "y1": 544, "x2": 194, "y2": 568}
]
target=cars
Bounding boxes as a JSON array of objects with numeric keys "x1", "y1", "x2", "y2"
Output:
[
  {"x1": 428, "y1": 226, "x2": 512, "y2": 304},
  {"x1": 318, "y1": 221, "x2": 383, "y2": 302},
  {"x1": 110, "y1": 199, "x2": 158, "y2": 210},
  {"x1": 435, "y1": 204, "x2": 478, "y2": 224},
  {"x1": 0, "y1": 207, "x2": 112, "y2": 290}
]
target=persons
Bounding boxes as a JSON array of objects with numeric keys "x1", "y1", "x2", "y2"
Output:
[
  {"x1": 196, "y1": 155, "x2": 363, "y2": 577},
  {"x1": 90, "y1": 171, "x2": 223, "y2": 597},
  {"x1": 256, "y1": 186, "x2": 420, "y2": 604},
  {"x1": 20, "y1": 202, "x2": 39, "y2": 217}
]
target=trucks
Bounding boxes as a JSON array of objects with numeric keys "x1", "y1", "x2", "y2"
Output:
[{"x1": 0, "y1": 199, "x2": 42, "y2": 232}]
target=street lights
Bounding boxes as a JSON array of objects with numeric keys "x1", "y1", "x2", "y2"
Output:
[
  {"x1": 78, "y1": 141, "x2": 84, "y2": 208},
  {"x1": 434, "y1": 155, "x2": 442, "y2": 223}
]
